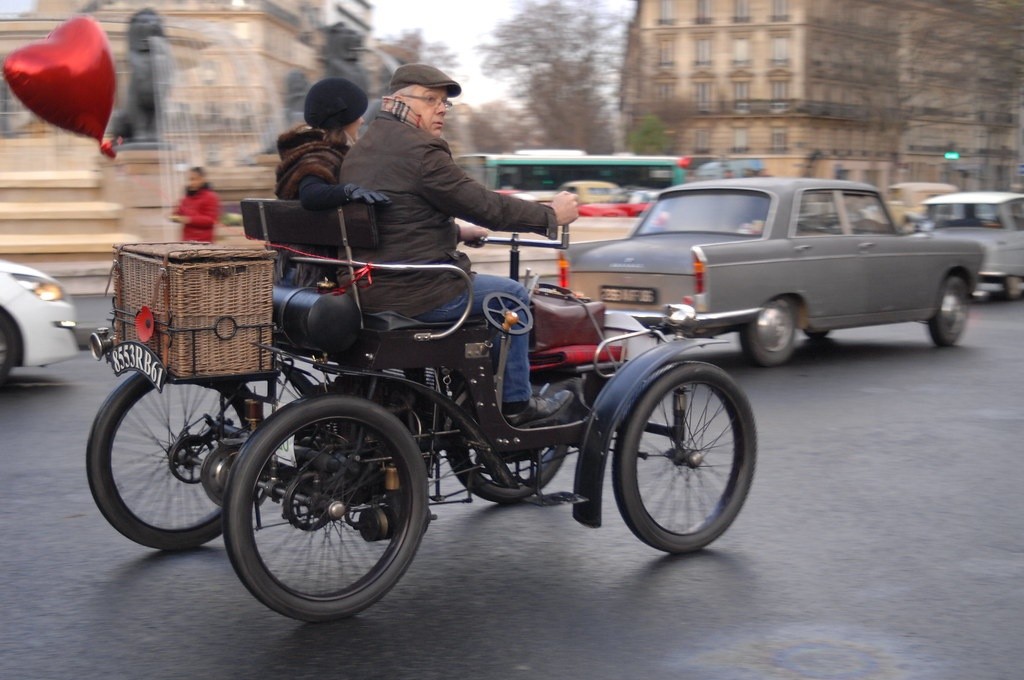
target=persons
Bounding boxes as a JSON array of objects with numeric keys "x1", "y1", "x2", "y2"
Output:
[
  {"x1": 273, "y1": 63, "x2": 579, "y2": 429},
  {"x1": 163, "y1": 165, "x2": 222, "y2": 245}
]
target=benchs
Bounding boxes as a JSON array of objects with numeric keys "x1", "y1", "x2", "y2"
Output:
[{"x1": 240, "y1": 197, "x2": 491, "y2": 371}]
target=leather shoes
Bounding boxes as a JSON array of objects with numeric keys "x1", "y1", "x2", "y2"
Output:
[{"x1": 505, "y1": 390, "x2": 574, "y2": 429}]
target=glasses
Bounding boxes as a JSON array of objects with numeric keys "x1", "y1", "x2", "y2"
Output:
[{"x1": 400, "y1": 93, "x2": 453, "y2": 111}]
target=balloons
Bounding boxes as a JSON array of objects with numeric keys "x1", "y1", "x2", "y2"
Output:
[{"x1": 1, "y1": 11, "x2": 121, "y2": 159}]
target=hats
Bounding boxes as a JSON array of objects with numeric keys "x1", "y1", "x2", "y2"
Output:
[
  {"x1": 390, "y1": 63, "x2": 461, "y2": 98},
  {"x1": 305, "y1": 78, "x2": 367, "y2": 128}
]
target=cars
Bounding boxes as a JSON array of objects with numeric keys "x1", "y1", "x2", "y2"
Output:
[
  {"x1": 887, "y1": 182, "x2": 956, "y2": 231},
  {"x1": 560, "y1": 178, "x2": 986, "y2": 368},
  {"x1": 491, "y1": 178, "x2": 659, "y2": 218},
  {"x1": 0, "y1": 258, "x2": 79, "y2": 390},
  {"x1": 914, "y1": 192, "x2": 1024, "y2": 302}
]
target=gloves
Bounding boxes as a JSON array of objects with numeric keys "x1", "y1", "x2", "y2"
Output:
[{"x1": 344, "y1": 183, "x2": 389, "y2": 205}]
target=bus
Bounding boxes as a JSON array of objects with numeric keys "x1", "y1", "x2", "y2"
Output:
[{"x1": 456, "y1": 147, "x2": 689, "y2": 194}]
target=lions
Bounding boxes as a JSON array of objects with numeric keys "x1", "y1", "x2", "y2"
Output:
[
  {"x1": 110, "y1": 8, "x2": 174, "y2": 144},
  {"x1": 319, "y1": 21, "x2": 369, "y2": 102}
]
